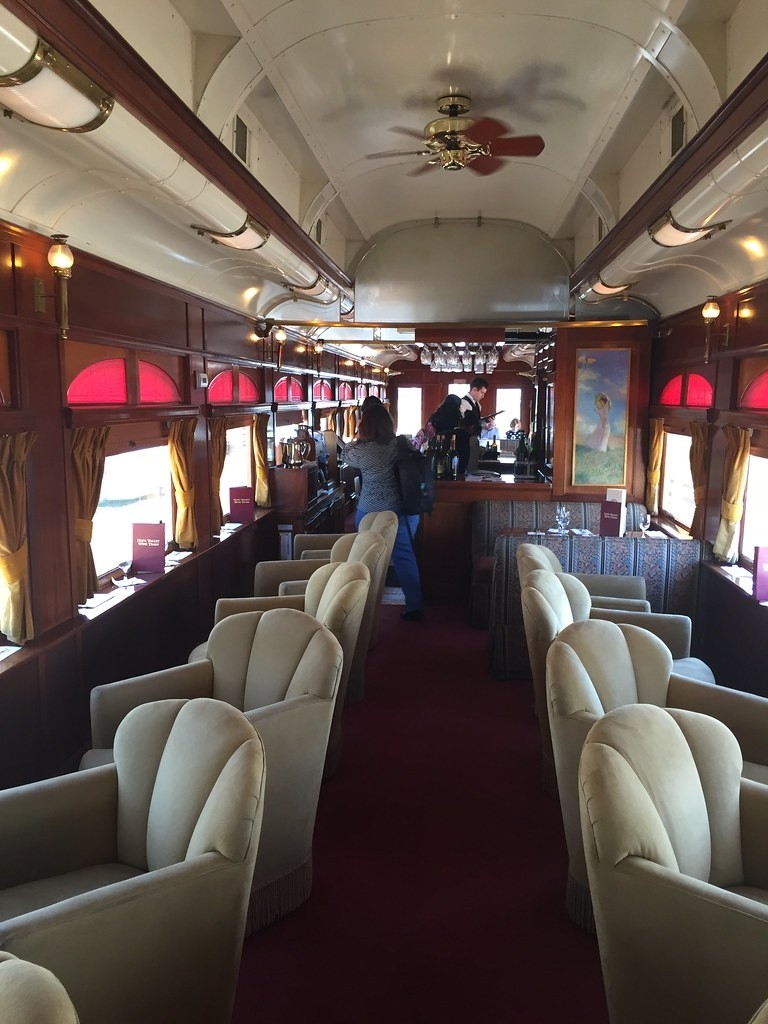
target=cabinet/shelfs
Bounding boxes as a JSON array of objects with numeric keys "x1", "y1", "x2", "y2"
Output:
[{"x1": 269, "y1": 465, "x2": 346, "y2": 534}]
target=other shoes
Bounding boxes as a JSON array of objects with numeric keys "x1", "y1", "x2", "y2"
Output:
[{"x1": 400, "y1": 609, "x2": 424, "y2": 621}]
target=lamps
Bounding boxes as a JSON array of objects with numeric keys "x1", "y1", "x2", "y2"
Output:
[
  {"x1": 314, "y1": 339, "x2": 325, "y2": 353},
  {"x1": 48, "y1": 232, "x2": 74, "y2": 281},
  {"x1": 699, "y1": 295, "x2": 721, "y2": 327},
  {"x1": 276, "y1": 325, "x2": 286, "y2": 345}
]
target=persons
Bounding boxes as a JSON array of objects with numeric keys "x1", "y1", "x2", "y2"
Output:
[
  {"x1": 425, "y1": 378, "x2": 495, "y2": 470},
  {"x1": 339, "y1": 404, "x2": 426, "y2": 624},
  {"x1": 355, "y1": 396, "x2": 390, "y2": 495}
]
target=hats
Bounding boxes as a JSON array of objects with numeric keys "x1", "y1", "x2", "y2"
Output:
[{"x1": 361, "y1": 396, "x2": 385, "y2": 410}]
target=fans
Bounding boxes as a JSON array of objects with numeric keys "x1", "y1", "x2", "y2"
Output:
[{"x1": 365, "y1": 93, "x2": 546, "y2": 178}]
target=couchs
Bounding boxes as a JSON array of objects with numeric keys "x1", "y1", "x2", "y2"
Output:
[
  {"x1": 73, "y1": 608, "x2": 344, "y2": 939},
  {"x1": 576, "y1": 706, "x2": 768, "y2": 1024},
  {"x1": 253, "y1": 511, "x2": 400, "y2": 639},
  {"x1": 0, "y1": 696, "x2": 266, "y2": 1024},
  {"x1": 187, "y1": 561, "x2": 372, "y2": 781},
  {"x1": 543, "y1": 619, "x2": 768, "y2": 936},
  {"x1": 521, "y1": 569, "x2": 716, "y2": 796},
  {"x1": 516, "y1": 544, "x2": 651, "y2": 613},
  {"x1": 214, "y1": 531, "x2": 387, "y2": 705}
]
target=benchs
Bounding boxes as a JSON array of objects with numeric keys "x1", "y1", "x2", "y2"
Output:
[
  {"x1": 486, "y1": 533, "x2": 703, "y2": 681},
  {"x1": 468, "y1": 499, "x2": 650, "y2": 626}
]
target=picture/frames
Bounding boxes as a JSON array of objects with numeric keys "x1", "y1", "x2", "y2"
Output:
[{"x1": 571, "y1": 346, "x2": 631, "y2": 487}]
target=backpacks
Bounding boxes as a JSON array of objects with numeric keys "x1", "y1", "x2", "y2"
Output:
[
  {"x1": 412, "y1": 412, "x2": 440, "y2": 451},
  {"x1": 398, "y1": 435, "x2": 434, "y2": 516}
]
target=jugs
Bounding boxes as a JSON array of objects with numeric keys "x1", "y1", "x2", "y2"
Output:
[
  {"x1": 290, "y1": 440, "x2": 311, "y2": 469},
  {"x1": 280, "y1": 442, "x2": 306, "y2": 468}
]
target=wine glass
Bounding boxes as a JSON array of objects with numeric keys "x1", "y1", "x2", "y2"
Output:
[
  {"x1": 558, "y1": 511, "x2": 571, "y2": 536},
  {"x1": 640, "y1": 514, "x2": 651, "y2": 538},
  {"x1": 556, "y1": 507, "x2": 565, "y2": 534}
]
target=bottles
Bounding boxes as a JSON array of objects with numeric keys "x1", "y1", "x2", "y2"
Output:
[
  {"x1": 435, "y1": 434, "x2": 459, "y2": 480},
  {"x1": 492, "y1": 435, "x2": 496, "y2": 460},
  {"x1": 486, "y1": 441, "x2": 489, "y2": 451}
]
